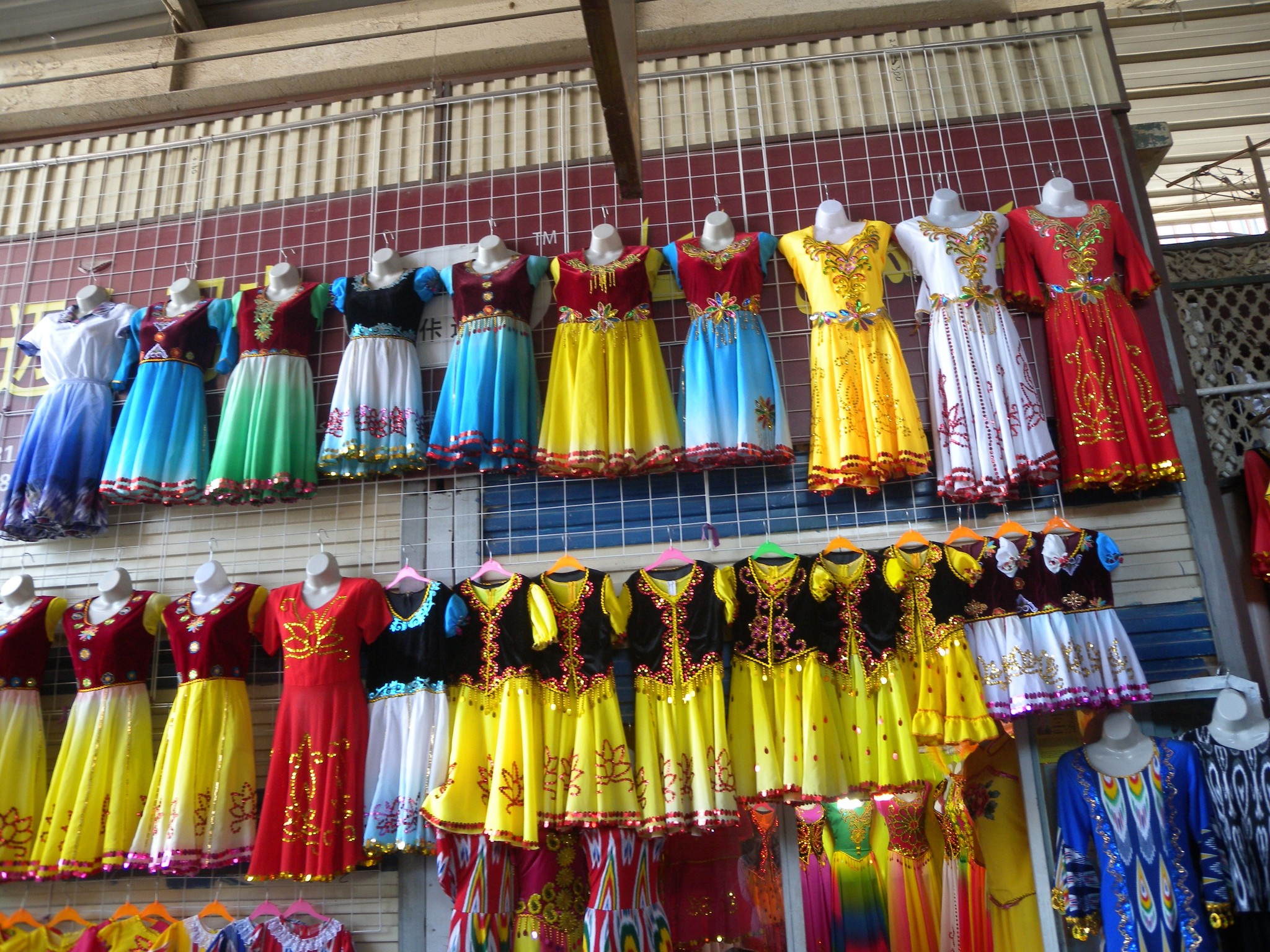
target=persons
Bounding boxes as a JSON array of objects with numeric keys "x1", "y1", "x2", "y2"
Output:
[
  {"x1": 1051, "y1": 689, "x2": 1270, "y2": 951},
  {"x1": 794, "y1": 719, "x2": 1045, "y2": 952},
  {"x1": 0, "y1": 177, "x2": 1187, "y2": 540},
  {"x1": 0, "y1": 552, "x2": 392, "y2": 881}
]
